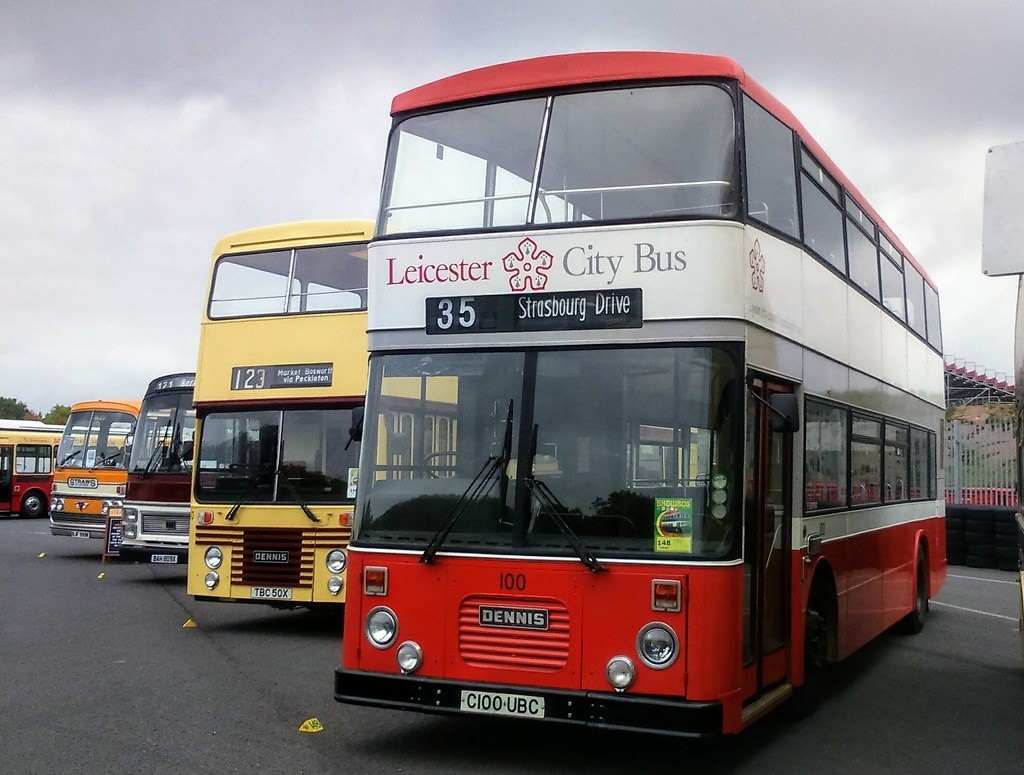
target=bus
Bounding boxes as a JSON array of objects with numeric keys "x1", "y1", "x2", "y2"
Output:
[
  {"x1": 117, "y1": 372, "x2": 357, "y2": 566},
  {"x1": 0, "y1": 426, "x2": 167, "y2": 518},
  {"x1": 329, "y1": 48, "x2": 949, "y2": 747},
  {"x1": 48, "y1": 400, "x2": 197, "y2": 540},
  {"x1": 186, "y1": 218, "x2": 701, "y2": 623}
]
